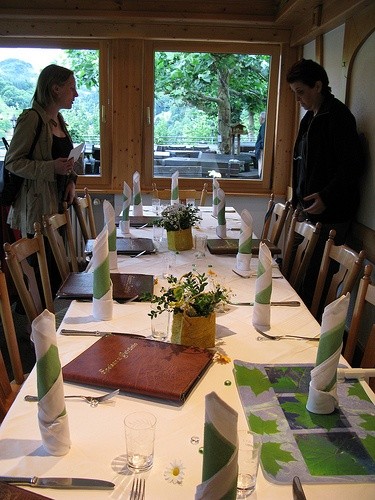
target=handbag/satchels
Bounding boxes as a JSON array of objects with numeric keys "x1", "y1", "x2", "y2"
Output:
[{"x1": 0, "y1": 108, "x2": 44, "y2": 208}]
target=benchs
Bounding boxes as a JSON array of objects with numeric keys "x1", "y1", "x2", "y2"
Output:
[{"x1": 82, "y1": 145, "x2": 260, "y2": 179}]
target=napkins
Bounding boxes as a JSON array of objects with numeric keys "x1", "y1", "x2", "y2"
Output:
[
  {"x1": 212, "y1": 177, "x2": 220, "y2": 218},
  {"x1": 31, "y1": 309, "x2": 71, "y2": 456},
  {"x1": 235, "y1": 208, "x2": 254, "y2": 271},
  {"x1": 195, "y1": 392, "x2": 238, "y2": 500},
  {"x1": 305, "y1": 292, "x2": 350, "y2": 414},
  {"x1": 216, "y1": 188, "x2": 227, "y2": 238},
  {"x1": 103, "y1": 200, "x2": 117, "y2": 269},
  {"x1": 132, "y1": 170, "x2": 143, "y2": 217},
  {"x1": 171, "y1": 170, "x2": 179, "y2": 205},
  {"x1": 252, "y1": 241, "x2": 273, "y2": 325},
  {"x1": 90, "y1": 223, "x2": 112, "y2": 321},
  {"x1": 119, "y1": 180, "x2": 131, "y2": 234}
]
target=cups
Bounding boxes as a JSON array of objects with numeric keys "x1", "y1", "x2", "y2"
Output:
[
  {"x1": 194, "y1": 234, "x2": 208, "y2": 257},
  {"x1": 186, "y1": 198, "x2": 196, "y2": 208},
  {"x1": 236, "y1": 430, "x2": 263, "y2": 498},
  {"x1": 162, "y1": 253, "x2": 177, "y2": 279},
  {"x1": 153, "y1": 223, "x2": 164, "y2": 242},
  {"x1": 151, "y1": 199, "x2": 161, "y2": 213},
  {"x1": 123, "y1": 412, "x2": 158, "y2": 473},
  {"x1": 151, "y1": 309, "x2": 171, "y2": 340}
]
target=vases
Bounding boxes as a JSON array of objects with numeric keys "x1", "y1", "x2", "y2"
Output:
[
  {"x1": 171, "y1": 312, "x2": 183, "y2": 344},
  {"x1": 180, "y1": 312, "x2": 216, "y2": 348},
  {"x1": 167, "y1": 227, "x2": 193, "y2": 251}
]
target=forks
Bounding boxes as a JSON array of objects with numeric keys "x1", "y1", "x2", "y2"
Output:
[
  {"x1": 256, "y1": 328, "x2": 320, "y2": 341},
  {"x1": 25, "y1": 388, "x2": 120, "y2": 403},
  {"x1": 231, "y1": 270, "x2": 283, "y2": 279},
  {"x1": 129, "y1": 477, "x2": 147, "y2": 499}
]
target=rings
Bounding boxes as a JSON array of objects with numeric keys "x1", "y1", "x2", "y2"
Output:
[{"x1": 67, "y1": 170, "x2": 71, "y2": 176}]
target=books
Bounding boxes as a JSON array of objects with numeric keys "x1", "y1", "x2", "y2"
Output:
[
  {"x1": 85, "y1": 238, "x2": 156, "y2": 254},
  {"x1": 62, "y1": 333, "x2": 215, "y2": 402},
  {"x1": 56, "y1": 271, "x2": 155, "y2": 301},
  {"x1": 206, "y1": 238, "x2": 281, "y2": 254},
  {"x1": 114, "y1": 215, "x2": 165, "y2": 228}
]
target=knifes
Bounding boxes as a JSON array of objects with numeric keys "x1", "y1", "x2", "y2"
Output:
[
  {"x1": 61, "y1": 329, "x2": 146, "y2": 339},
  {"x1": 228, "y1": 300, "x2": 301, "y2": 306},
  {"x1": 293, "y1": 476, "x2": 307, "y2": 500},
  {"x1": 0, "y1": 475, "x2": 116, "y2": 490}
]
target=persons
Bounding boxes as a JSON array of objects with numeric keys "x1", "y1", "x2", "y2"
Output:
[
  {"x1": 4, "y1": 64, "x2": 78, "y2": 341},
  {"x1": 255, "y1": 112, "x2": 266, "y2": 177},
  {"x1": 287, "y1": 60, "x2": 364, "y2": 326}
]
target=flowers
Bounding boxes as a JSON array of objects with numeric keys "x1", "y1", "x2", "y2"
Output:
[
  {"x1": 148, "y1": 203, "x2": 204, "y2": 232},
  {"x1": 132, "y1": 266, "x2": 236, "y2": 318}
]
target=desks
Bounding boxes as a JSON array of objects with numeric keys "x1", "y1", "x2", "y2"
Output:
[{"x1": 0, "y1": 205, "x2": 375, "y2": 500}]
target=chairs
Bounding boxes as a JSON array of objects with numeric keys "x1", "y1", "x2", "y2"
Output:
[{"x1": 0, "y1": 187, "x2": 375, "y2": 426}]
